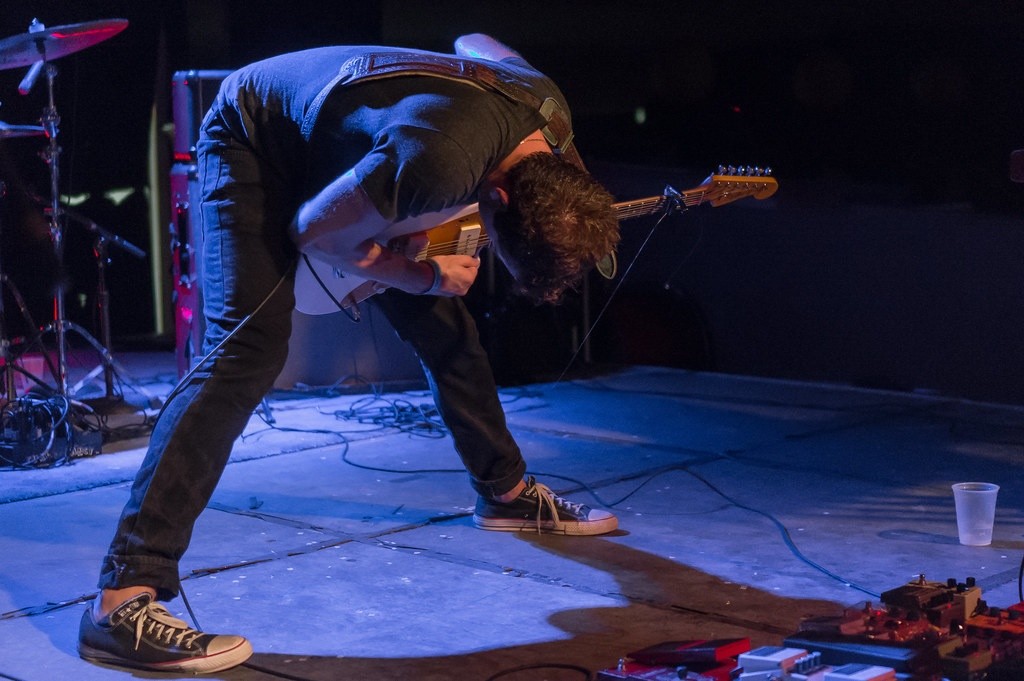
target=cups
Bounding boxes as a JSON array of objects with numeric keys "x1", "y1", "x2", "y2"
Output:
[{"x1": 951, "y1": 482, "x2": 1000, "y2": 546}]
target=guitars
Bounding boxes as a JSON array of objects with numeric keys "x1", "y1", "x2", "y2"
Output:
[{"x1": 295, "y1": 164, "x2": 783, "y2": 314}]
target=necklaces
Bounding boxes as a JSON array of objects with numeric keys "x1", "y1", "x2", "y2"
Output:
[{"x1": 521, "y1": 138, "x2": 546, "y2": 144}]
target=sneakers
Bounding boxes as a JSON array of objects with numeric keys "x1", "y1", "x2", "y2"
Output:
[
  {"x1": 77, "y1": 592, "x2": 253, "y2": 676},
  {"x1": 473, "y1": 475, "x2": 618, "y2": 535}
]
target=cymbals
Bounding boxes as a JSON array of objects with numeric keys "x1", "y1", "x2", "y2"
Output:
[
  {"x1": 1, "y1": 120, "x2": 59, "y2": 134},
  {"x1": 0, "y1": 18, "x2": 129, "y2": 69}
]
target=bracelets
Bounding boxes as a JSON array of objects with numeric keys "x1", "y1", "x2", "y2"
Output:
[{"x1": 418, "y1": 258, "x2": 442, "y2": 295}]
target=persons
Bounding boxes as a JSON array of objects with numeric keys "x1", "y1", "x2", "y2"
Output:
[{"x1": 78, "y1": 33, "x2": 619, "y2": 675}]
